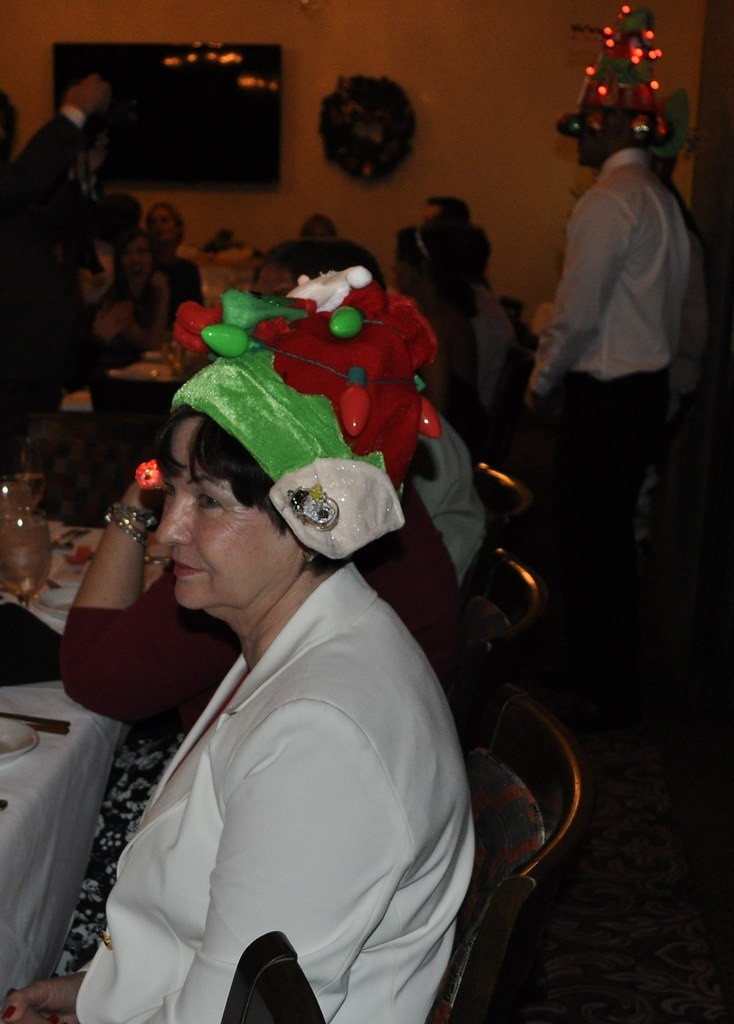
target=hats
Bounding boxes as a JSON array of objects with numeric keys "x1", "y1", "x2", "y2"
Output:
[
  {"x1": 555, "y1": 3, "x2": 689, "y2": 156},
  {"x1": 171, "y1": 266, "x2": 442, "y2": 562},
  {"x1": 397, "y1": 220, "x2": 493, "y2": 308}
]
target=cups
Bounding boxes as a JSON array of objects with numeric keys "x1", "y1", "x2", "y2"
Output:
[{"x1": 0, "y1": 480, "x2": 33, "y2": 518}]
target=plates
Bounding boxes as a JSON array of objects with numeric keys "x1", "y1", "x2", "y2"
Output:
[
  {"x1": 0, "y1": 717, "x2": 39, "y2": 759},
  {"x1": 37, "y1": 587, "x2": 80, "y2": 621}
]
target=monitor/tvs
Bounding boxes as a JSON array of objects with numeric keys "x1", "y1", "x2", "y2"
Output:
[{"x1": 54, "y1": 42, "x2": 282, "y2": 190}]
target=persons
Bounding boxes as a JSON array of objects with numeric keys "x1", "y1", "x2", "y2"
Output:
[
  {"x1": 0, "y1": 237, "x2": 492, "y2": 1024},
  {"x1": 0, "y1": 77, "x2": 202, "y2": 410},
  {"x1": 299, "y1": 197, "x2": 518, "y2": 423},
  {"x1": 522, "y1": 105, "x2": 710, "y2": 731}
]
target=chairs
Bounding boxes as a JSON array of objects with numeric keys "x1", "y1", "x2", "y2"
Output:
[
  {"x1": 423, "y1": 682, "x2": 595, "y2": 1024},
  {"x1": 459, "y1": 462, "x2": 550, "y2": 746}
]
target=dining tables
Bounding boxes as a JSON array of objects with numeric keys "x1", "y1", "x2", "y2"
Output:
[{"x1": 0, "y1": 528, "x2": 172, "y2": 1009}]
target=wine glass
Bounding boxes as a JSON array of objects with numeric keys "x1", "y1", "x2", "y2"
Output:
[{"x1": 0, "y1": 507, "x2": 51, "y2": 610}]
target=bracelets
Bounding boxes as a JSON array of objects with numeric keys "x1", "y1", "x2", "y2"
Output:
[
  {"x1": 104, "y1": 501, "x2": 155, "y2": 547},
  {"x1": 141, "y1": 551, "x2": 172, "y2": 566}
]
